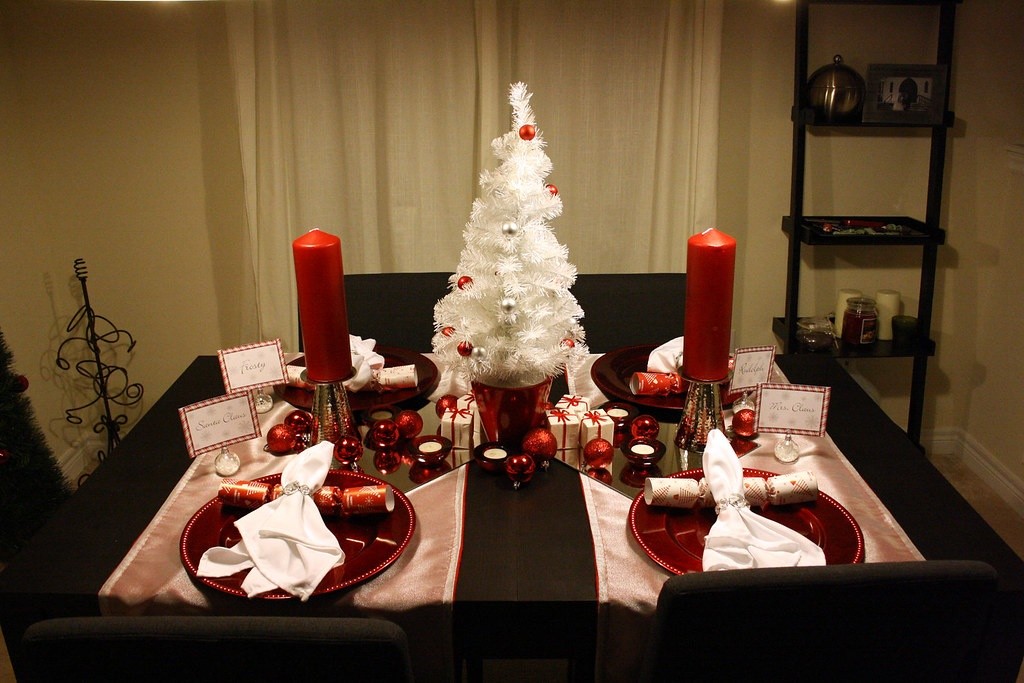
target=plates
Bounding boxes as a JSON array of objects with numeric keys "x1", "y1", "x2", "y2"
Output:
[
  {"x1": 183, "y1": 468, "x2": 417, "y2": 600},
  {"x1": 627, "y1": 468, "x2": 864, "y2": 582},
  {"x1": 590, "y1": 344, "x2": 763, "y2": 412},
  {"x1": 274, "y1": 345, "x2": 440, "y2": 421}
]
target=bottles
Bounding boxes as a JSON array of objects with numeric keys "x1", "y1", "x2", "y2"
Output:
[{"x1": 840, "y1": 297, "x2": 880, "y2": 351}]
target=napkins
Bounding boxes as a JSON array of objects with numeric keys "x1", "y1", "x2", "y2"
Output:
[
  {"x1": 700, "y1": 429, "x2": 826, "y2": 576},
  {"x1": 341, "y1": 332, "x2": 385, "y2": 392},
  {"x1": 194, "y1": 440, "x2": 346, "y2": 601},
  {"x1": 646, "y1": 335, "x2": 685, "y2": 374}
]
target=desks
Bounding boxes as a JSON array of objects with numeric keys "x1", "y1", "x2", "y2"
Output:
[{"x1": 0, "y1": 350, "x2": 1023, "y2": 683}]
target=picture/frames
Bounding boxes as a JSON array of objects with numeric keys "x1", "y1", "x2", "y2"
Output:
[{"x1": 861, "y1": 63, "x2": 939, "y2": 125}]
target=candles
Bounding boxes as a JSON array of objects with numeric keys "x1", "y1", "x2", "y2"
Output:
[
  {"x1": 681, "y1": 225, "x2": 737, "y2": 382},
  {"x1": 292, "y1": 228, "x2": 353, "y2": 383}
]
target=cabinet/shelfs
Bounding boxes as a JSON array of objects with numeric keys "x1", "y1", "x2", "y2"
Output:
[{"x1": 772, "y1": 1, "x2": 958, "y2": 458}]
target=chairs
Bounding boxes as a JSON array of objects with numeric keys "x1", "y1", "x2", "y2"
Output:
[
  {"x1": 20, "y1": 616, "x2": 417, "y2": 683},
  {"x1": 643, "y1": 558, "x2": 1011, "y2": 683},
  {"x1": 297, "y1": 271, "x2": 462, "y2": 351},
  {"x1": 564, "y1": 269, "x2": 690, "y2": 355}
]
